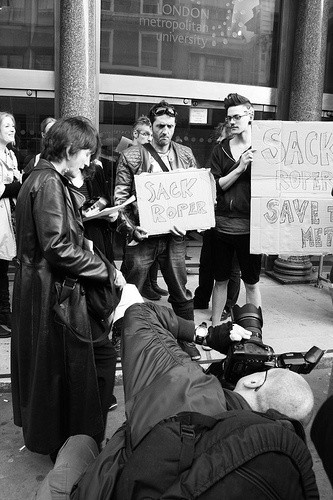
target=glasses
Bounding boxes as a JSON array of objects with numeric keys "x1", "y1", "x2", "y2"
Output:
[
  {"x1": 225, "y1": 115, "x2": 249, "y2": 121},
  {"x1": 140, "y1": 132, "x2": 150, "y2": 136},
  {"x1": 153, "y1": 106, "x2": 177, "y2": 117}
]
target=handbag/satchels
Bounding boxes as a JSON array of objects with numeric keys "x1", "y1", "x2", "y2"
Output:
[{"x1": 55, "y1": 242, "x2": 122, "y2": 342}]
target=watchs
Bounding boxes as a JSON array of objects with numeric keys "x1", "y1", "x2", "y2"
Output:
[{"x1": 195, "y1": 322, "x2": 208, "y2": 345}]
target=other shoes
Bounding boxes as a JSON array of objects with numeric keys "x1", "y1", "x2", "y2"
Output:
[
  {"x1": 141, "y1": 289, "x2": 161, "y2": 300},
  {"x1": 179, "y1": 341, "x2": 199, "y2": 360},
  {"x1": 210, "y1": 312, "x2": 231, "y2": 320},
  {"x1": 0, "y1": 326, "x2": 11, "y2": 337},
  {"x1": 193, "y1": 296, "x2": 208, "y2": 309},
  {"x1": 110, "y1": 395, "x2": 118, "y2": 409},
  {"x1": 152, "y1": 285, "x2": 167, "y2": 295}
]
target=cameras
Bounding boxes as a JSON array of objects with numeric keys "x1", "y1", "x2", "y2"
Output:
[{"x1": 223, "y1": 303, "x2": 325, "y2": 385}]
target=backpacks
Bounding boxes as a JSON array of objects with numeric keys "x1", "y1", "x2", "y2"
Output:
[{"x1": 109, "y1": 389, "x2": 320, "y2": 500}]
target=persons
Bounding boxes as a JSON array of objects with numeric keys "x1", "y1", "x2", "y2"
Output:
[
  {"x1": 37, "y1": 302, "x2": 320, "y2": 500},
  {"x1": 0, "y1": 111, "x2": 118, "y2": 412},
  {"x1": 192, "y1": 93, "x2": 261, "y2": 351},
  {"x1": 114, "y1": 101, "x2": 200, "y2": 358},
  {"x1": 11, "y1": 116, "x2": 126, "y2": 464}
]
